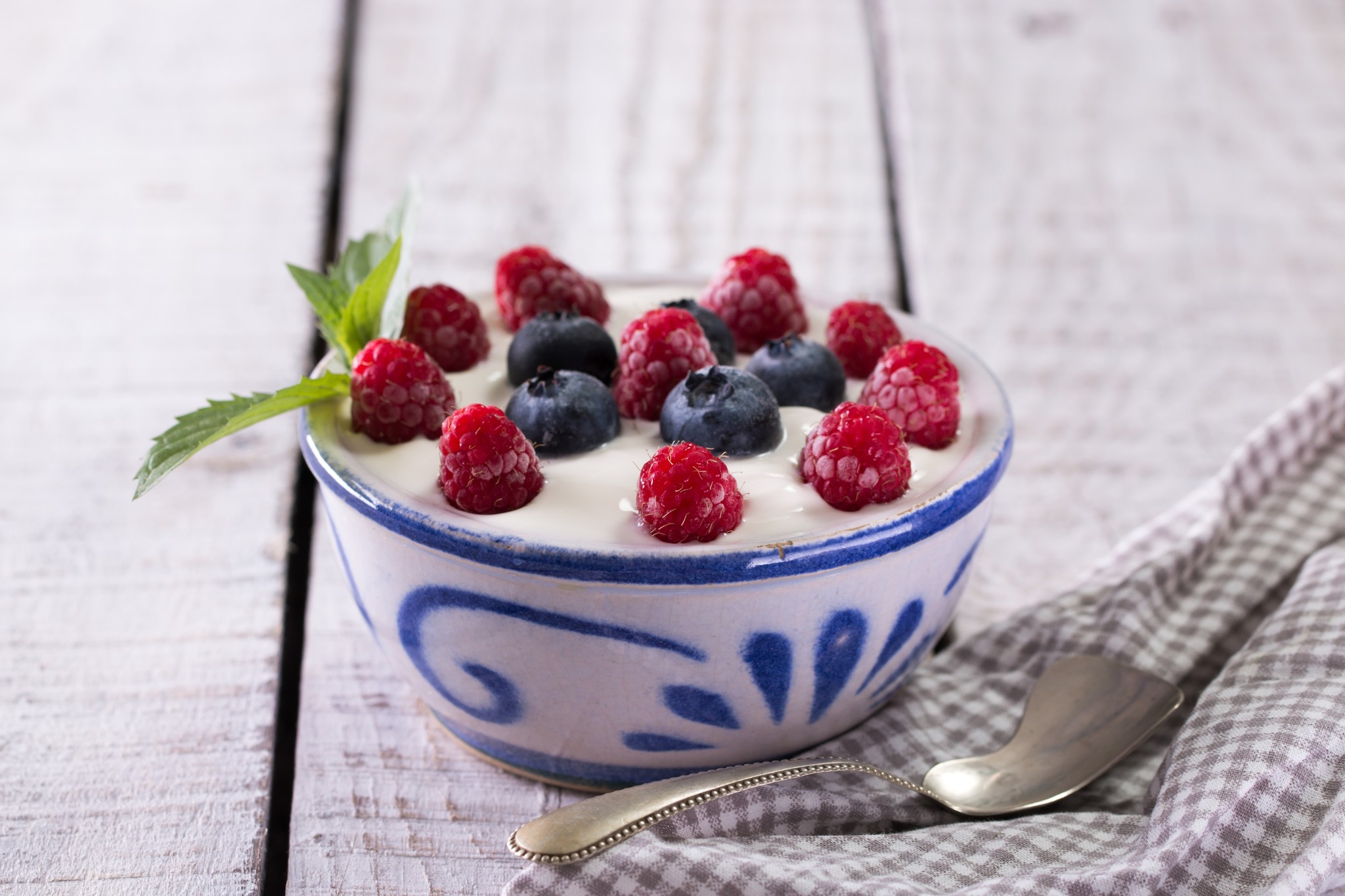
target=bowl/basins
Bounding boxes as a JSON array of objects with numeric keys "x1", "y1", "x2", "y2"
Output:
[{"x1": 296, "y1": 275, "x2": 1015, "y2": 795}]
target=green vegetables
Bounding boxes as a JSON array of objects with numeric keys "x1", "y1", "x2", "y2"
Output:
[{"x1": 133, "y1": 197, "x2": 410, "y2": 500}]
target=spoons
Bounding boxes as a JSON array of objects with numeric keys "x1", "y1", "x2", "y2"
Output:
[{"x1": 508, "y1": 654, "x2": 1185, "y2": 865}]
